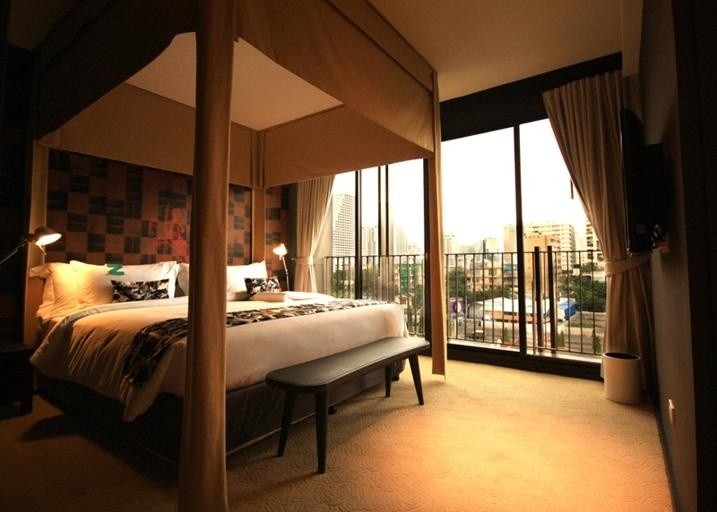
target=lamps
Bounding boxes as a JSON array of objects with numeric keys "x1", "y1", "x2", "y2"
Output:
[
  {"x1": 272, "y1": 243, "x2": 289, "y2": 291},
  {"x1": 0, "y1": 226, "x2": 62, "y2": 265}
]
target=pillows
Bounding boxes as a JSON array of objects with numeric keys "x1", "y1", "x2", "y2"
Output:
[
  {"x1": 69, "y1": 260, "x2": 180, "y2": 278},
  {"x1": 178, "y1": 260, "x2": 267, "y2": 301},
  {"x1": 29, "y1": 260, "x2": 177, "y2": 319},
  {"x1": 110, "y1": 278, "x2": 169, "y2": 303},
  {"x1": 244, "y1": 274, "x2": 281, "y2": 300}
]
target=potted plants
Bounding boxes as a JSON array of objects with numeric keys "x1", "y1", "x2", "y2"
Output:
[{"x1": 499, "y1": 328, "x2": 511, "y2": 347}]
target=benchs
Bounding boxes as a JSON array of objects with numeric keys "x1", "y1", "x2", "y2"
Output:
[{"x1": 265, "y1": 336, "x2": 431, "y2": 474}]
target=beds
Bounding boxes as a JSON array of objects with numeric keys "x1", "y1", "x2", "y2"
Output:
[{"x1": 28, "y1": 259, "x2": 408, "y2": 468}]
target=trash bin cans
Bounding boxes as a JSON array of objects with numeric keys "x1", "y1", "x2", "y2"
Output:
[{"x1": 602, "y1": 352, "x2": 644, "y2": 405}]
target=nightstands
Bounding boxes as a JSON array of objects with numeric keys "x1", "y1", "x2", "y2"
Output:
[{"x1": 0, "y1": 337, "x2": 37, "y2": 420}]
